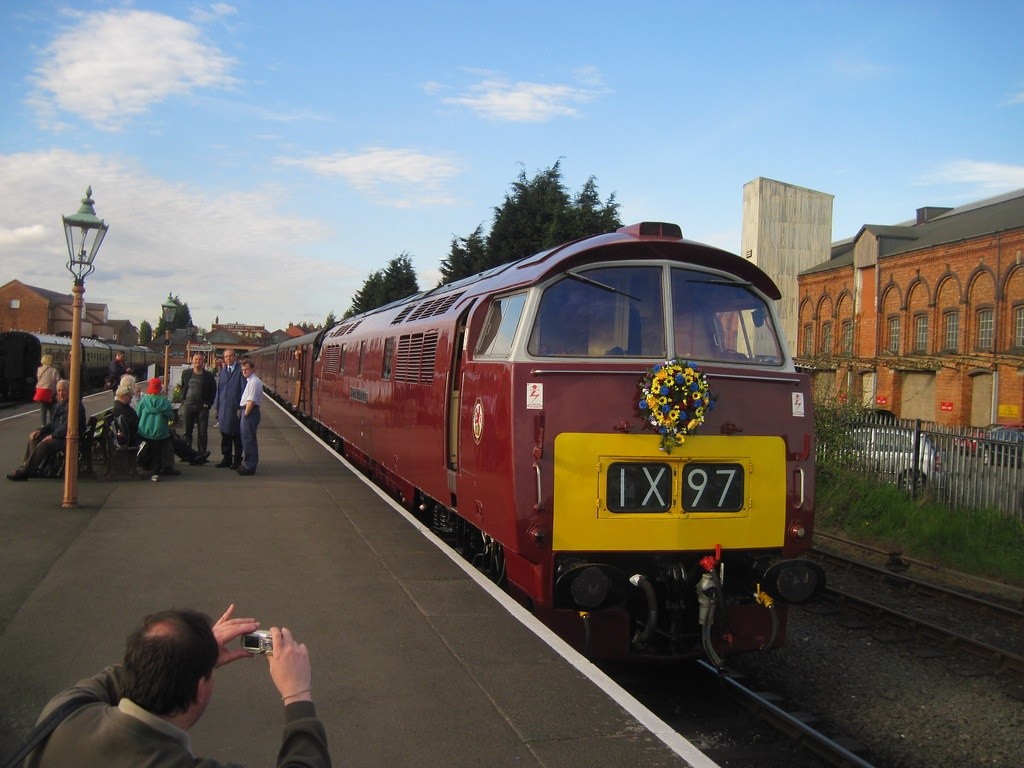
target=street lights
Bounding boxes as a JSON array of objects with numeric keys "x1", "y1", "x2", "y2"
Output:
[
  {"x1": 62, "y1": 186, "x2": 109, "y2": 511},
  {"x1": 185, "y1": 320, "x2": 197, "y2": 365},
  {"x1": 197, "y1": 329, "x2": 217, "y2": 371},
  {"x1": 161, "y1": 291, "x2": 178, "y2": 390}
]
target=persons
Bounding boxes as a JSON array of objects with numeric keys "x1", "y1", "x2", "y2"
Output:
[
  {"x1": 6, "y1": 374, "x2": 211, "y2": 481},
  {"x1": 59, "y1": 351, "x2": 83, "y2": 400},
  {"x1": 35, "y1": 355, "x2": 59, "y2": 426},
  {"x1": 22, "y1": 603, "x2": 332, "y2": 768},
  {"x1": 180, "y1": 351, "x2": 217, "y2": 463},
  {"x1": 215, "y1": 350, "x2": 248, "y2": 470},
  {"x1": 235, "y1": 358, "x2": 263, "y2": 476},
  {"x1": 109, "y1": 350, "x2": 133, "y2": 397}
]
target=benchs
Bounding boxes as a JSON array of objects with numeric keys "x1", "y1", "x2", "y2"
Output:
[
  {"x1": 167, "y1": 401, "x2": 182, "y2": 422},
  {"x1": 44, "y1": 407, "x2": 141, "y2": 480}
]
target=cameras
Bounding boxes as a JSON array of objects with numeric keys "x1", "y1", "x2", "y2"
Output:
[{"x1": 242, "y1": 630, "x2": 274, "y2": 654}]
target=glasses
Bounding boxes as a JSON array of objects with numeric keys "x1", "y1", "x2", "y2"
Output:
[{"x1": 123, "y1": 394, "x2": 131, "y2": 397}]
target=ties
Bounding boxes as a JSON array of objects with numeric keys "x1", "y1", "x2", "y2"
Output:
[{"x1": 228, "y1": 368, "x2": 231, "y2": 375}]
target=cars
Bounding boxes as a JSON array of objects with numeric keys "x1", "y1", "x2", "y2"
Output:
[{"x1": 814, "y1": 403, "x2": 1024, "y2": 497}]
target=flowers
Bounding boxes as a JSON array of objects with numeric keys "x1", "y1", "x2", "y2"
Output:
[{"x1": 637, "y1": 355, "x2": 720, "y2": 453}]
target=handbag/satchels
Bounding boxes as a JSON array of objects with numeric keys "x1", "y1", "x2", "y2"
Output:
[{"x1": 33, "y1": 388, "x2": 53, "y2": 403}]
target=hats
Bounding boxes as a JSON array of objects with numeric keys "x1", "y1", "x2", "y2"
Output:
[{"x1": 146, "y1": 378, "x2": 162, "y2": 394}]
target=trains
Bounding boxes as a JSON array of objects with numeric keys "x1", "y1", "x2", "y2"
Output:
[
  {"x1": 241, "y1": 221, "x2": 827, "y2": 661},
  {"x1": 0, "y1": 328, "x2": 156, "y2": 403}
]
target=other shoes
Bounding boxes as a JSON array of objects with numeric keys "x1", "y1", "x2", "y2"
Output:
[
  {"x1": 214, "y1": 455, "x2": 233, "y2": 467},
  {"x1": 7, "y1": 466, "x2": 28, "y2": 481},
  {"x1": 213, "y1": 422, "x2": 220, "y2": 428},
  {"x1": 230, "y1": 456, "x2": 243, "y2": 470},
  {"x1": 137, "y1": 441, "x2": 153, "y2": 458},
  {"x1": 237, "y1": 466, "x2": 256, "y2": 476},
  {"x1": 189, "y1": 449, "x2": 211, "y2": 466},
  {"x1": 162, "y1": 469, "x2": 181, "y2": 475}
]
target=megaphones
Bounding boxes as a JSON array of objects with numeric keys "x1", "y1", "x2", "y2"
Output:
[{"x1": 164, "y1": 340, "x2": 171, "y2": 347}]
